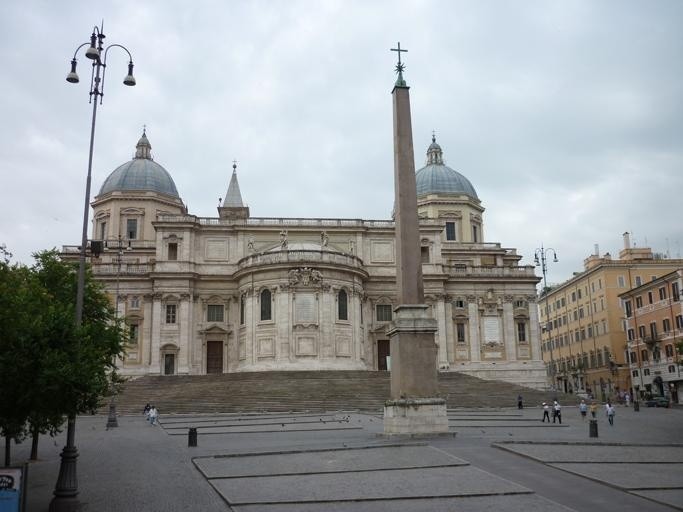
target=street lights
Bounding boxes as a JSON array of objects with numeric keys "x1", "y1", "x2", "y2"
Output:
[
  {"x1": 42, "y1": 21, "x2": 137, "y2": 510},
  {"x1": 533, "y1": 241, "x2": 559, "y2": 406},
  {"x1": 102, "y1": 230, "x2": 133, "y2": 428}
]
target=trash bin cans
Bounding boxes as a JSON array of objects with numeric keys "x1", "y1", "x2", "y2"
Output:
[
  {"x1": 634, "y1": 401, "x2": 639, "y2": 411},
  {"x1": 590, "y1": 420, "x2": 598, "y2": 437},
  {"x1": 188, "y1": 428, "x2": 197, "y2": 447}
]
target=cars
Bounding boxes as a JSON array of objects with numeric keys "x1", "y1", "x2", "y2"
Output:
[{"x1": 567, "y1": 388, "x2": 670, "y2": 408}]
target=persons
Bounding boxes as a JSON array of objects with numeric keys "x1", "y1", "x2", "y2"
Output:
[
  {"x1": 589, "y1": 402, "x2": 597, "y2": 418},
  {"x1": 516, "y1": 394, "x2": 523, "y2": 408},
  {"x1": 311, "y1": 269, "x2": 322, "y2": 282},
  {"x1": 290, "y1": 269, "x2": 299, "y2": 286},
  {"x1": 624, "y1": 393, "x2": 629, "y2": 406},
  {"x1": 149, "y1": 406, "x2": 157, "y2": 425},
  {"x1": 143, "y1": 403, "x2": 150, "y2": 420},
  {"x1": 301, "y1": 267, "x2": 309, "y2": 285},
  {"x1": 541, "y1": 403, "x2": 549, "y2": 422},
  {"x1": 606, "y1": 404, "x2": 614, "y2": 424},
  {"x1": 578, "y1": 400, "x2": 587, "y2": 422},
  {"x1": 552, "y1": 400, "x2": 561, "y2": 423}
]
